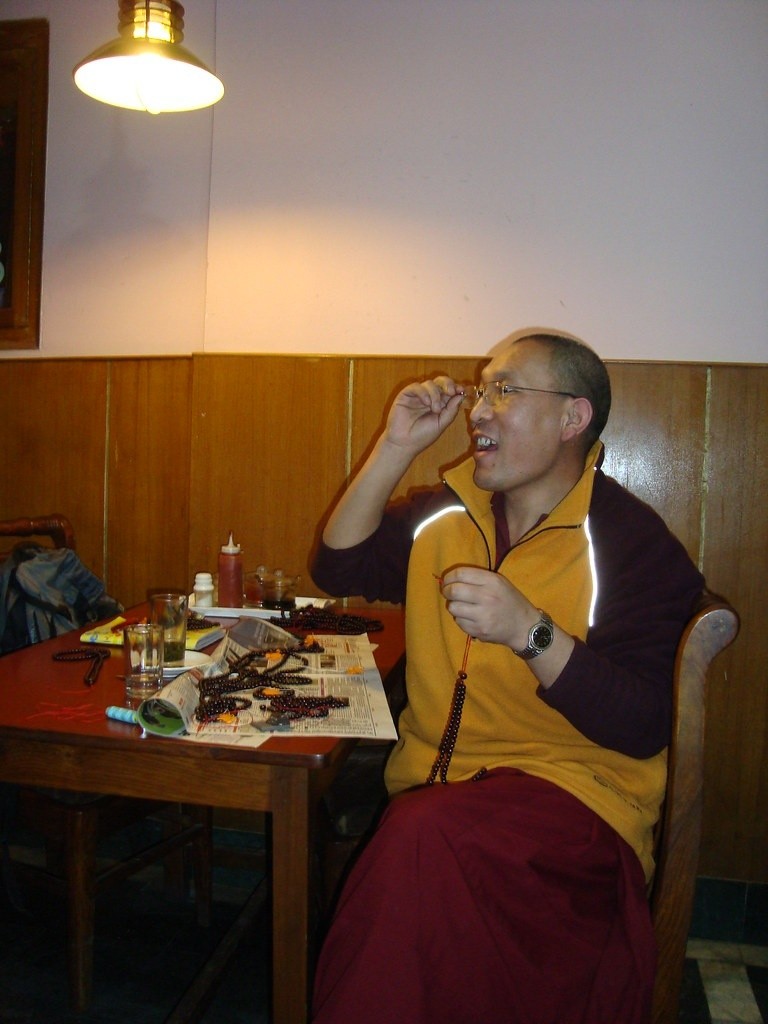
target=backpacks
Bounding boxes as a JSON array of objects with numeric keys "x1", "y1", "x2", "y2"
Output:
[{"x1": 0, "y1": 539, "x2": 123, "y2": 806}]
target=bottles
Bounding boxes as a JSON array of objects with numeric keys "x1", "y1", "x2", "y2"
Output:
[
  {"x1": 244, "y1": 566, "x2": 298, "y2": 610},
  {"x1": 194, "y1": 572, "x2": 215, "y2": 607},
  {"x1": 218, "y1": 533, "x2": 243, "y2": 608}
]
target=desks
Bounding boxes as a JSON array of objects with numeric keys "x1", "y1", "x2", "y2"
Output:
[{"x1": 0, "y1": 581, "x2": 404, "y2": 1023}]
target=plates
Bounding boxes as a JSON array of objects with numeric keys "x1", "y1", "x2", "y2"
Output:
[{"x1": 163, "y1": 650, "x2": 209, "y2": 677}]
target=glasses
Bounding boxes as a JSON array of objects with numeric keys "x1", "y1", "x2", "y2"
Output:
[{"x1": 458, "y1": 384, "x2": 580, "y2": 408}]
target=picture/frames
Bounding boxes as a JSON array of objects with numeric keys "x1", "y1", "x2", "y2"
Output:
[{"x1": 0, "y1": 20, "x2": 50, "y2": 349}]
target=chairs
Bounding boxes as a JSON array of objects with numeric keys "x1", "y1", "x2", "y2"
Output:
[
  {"x1": 323, "y1": 576, "x2": 741, "y2": 1024},
  {"x1": 0, "y1": 515, "x2": 217, "y2": 1015}
]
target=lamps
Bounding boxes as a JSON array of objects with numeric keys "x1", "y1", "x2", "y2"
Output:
[{"x1": 72, "y1": 0, "x2": 225, "y2": 118}]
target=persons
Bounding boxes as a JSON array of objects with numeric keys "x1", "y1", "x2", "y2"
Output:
[{"x1": 312, "y1": 333, "x2": 705, "y2": 1024}]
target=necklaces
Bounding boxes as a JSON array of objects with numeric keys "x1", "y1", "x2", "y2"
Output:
[
  {"x1": 148, "y1": 611, "x2": 220, "y2": 628},
  {"x1": 193, "y1": 649, "x2": 344, "y2": 723},
  {"x1": 54, "y1": 649, "x2": 111, "y2": 683},
  {"x1": 427, "y1": 577, "x2": 488, "y2": 786},
  {"x1": 268, "y1": 604, "x2": 384, "y2": 635}
]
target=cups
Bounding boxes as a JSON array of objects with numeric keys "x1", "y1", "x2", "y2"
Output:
[
  {"x1": 151, "y1": 594, "x2": 188, "y2": 668},
  {"x1": 124, "y1": 624, "x2": 164, "y2": 707}
]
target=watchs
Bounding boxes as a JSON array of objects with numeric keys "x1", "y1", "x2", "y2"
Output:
[{"x1": 513, "y1": 608, "x2": 554, "y2": 659}]
